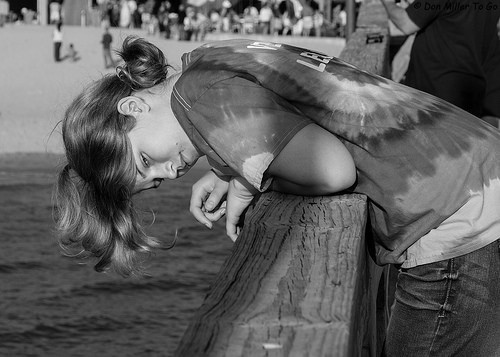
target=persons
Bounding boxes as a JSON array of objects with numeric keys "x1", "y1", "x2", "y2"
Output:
[
  {"x1": 52, "y1": 20, "x2": 64, "y2": 62},
  {"x1": 100, "y1": 25, "x2": 116, "y2": 68},
  {"x1": 68, "y1": 43, "x2": 81, "y2": 62},
  {"x1": 0, "y1": 0, "x2": 363, "y2": 45},
  {"x1": 48, "y1": 34, "x2": 500, "y2": 357},
  {"x1": 380, "y1": 0, "x2": 499, "y2": 134}
]
